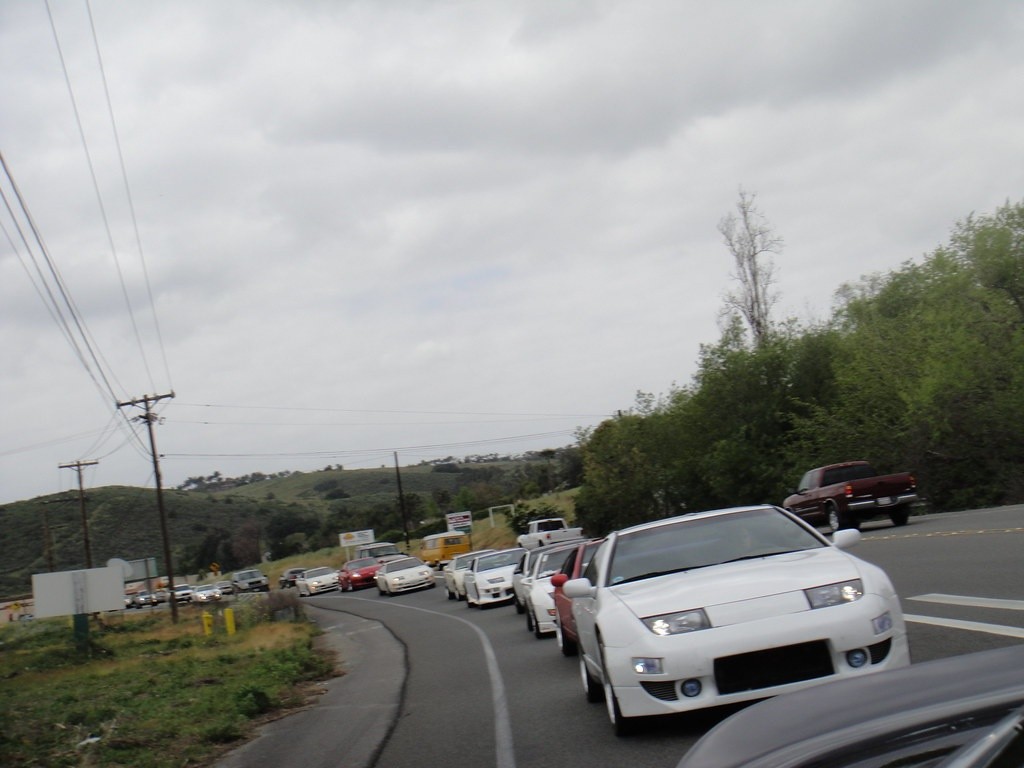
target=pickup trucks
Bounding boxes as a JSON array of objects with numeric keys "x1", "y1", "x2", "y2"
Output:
[
  {"x1": 516, "y1": 517, "x2": 583, "y2": 550},
  {"x1": 782, "y1": 460, "x2": 918, "y2": 533}
]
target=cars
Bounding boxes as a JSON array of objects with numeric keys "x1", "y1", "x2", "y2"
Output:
[
  {"x1": 443, "y1": 549, "x2": 498, "y2": 602},
  {"x1": 278, "y1": 567, "x2": 310, "y2": 589},
  {"x1": 560, "y1": 501, "x2": 912, "y2": 735},
  {"x1": 510, "y1": 539, "x2": 586, "y2": 615},
  {"x1": 295, "y1": 566, "x2": 340, "y2": 597},
  {"x1": 463, "y1": 547, "x2": 529, "y2": 609},
  {"x1": 122, "y1": 579, "x2": 233, "y2": 610},
  {"x1": 669, "y1": 642, "x2": 1024, "y2": 768},
  {"x1": 371, "y1": 556, "x2": 437, "y2": 597},
  {"x1": 549, "y1": 537, "x2": 609, "y2": 657},
  {"x1": 519, "y1": 538, "x2": 600, "y2": 638},
  {"x1": 337, "y1": 556, "x2": 384, "y2": 592}
]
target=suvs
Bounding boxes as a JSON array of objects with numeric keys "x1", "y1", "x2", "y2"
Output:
[
  {"x1": 352, "y1": 542, "x2": 410, "y2": 565},
  {"x1": 230, "y1": 568, "x2": 271, "y2": 595}
]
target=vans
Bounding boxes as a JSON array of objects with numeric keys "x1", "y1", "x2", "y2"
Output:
[{"x1": 412, "y1": 531, "x2": 471, "y2": 571}]
